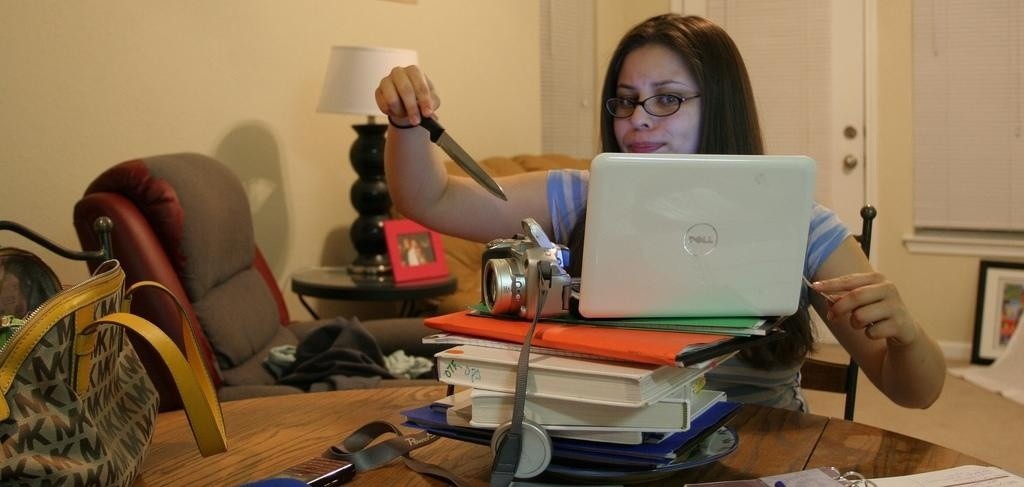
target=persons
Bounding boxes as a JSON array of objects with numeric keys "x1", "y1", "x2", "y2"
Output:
[
  {"x1": 375, "y1": 11, "x2": 946, "y2": 415},
  {"x1": 415, "y1": 245, "x2": 428, "y2": 264},
  {"x1": 398, "y1": 237, "x2": 408, "y2": 266},
  {"x1": 407, "y1": 239, "x2": 419, "y2": 265}
]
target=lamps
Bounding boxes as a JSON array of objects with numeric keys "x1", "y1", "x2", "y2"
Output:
[{"x1": 314, "y1": 45, "x2": 419, "y2": 284}]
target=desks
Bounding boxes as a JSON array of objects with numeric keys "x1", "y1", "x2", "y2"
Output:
[
  {"x1": 133, "y1": 383, "x2": 1022, "y2": 487},
  {"x1": 290, "y1": 270, "x2": 458, "y2": 321}
]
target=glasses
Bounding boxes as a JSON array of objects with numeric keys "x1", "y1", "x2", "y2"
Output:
[{"x1": 601, "y1": 91, "x2": 699, "y2": 118}]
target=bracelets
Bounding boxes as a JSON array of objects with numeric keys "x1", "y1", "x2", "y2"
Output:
[{"x1": 387, "y1": 114, "x2": 417, "y2": 128}]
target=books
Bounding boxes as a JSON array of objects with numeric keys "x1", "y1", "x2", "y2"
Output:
[
  {"x1": 685, "y1": 464, "x2": 878, "y2": 486},
  {"x1": 418, "y1": 315, "x2": 784, "y2": 463}
]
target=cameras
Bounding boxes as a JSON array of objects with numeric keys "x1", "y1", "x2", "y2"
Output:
[{"x1": 480, "y1": 216, "x2": 571, "y2": 319}]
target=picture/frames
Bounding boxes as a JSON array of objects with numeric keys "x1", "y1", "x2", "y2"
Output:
[
  {"x1": 971, "y1": 260, "x2": 1024, "y2": 366},
  {"x1": 383, "y1": 219, "x2": 449, "y2": 283}
]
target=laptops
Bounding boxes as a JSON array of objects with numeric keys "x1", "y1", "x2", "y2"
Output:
[{"x1": 568, "y1": 152, "x2": 816, "y2": 320}]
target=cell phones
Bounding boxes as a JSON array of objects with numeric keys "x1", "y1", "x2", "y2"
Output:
[{"x1": 267, "y1": 455, "x2": 356, "y2": 486}]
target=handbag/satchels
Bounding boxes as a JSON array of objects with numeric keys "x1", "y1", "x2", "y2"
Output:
[{"x1": 0, "y1": 248, "x2": 228, "y2": 485}]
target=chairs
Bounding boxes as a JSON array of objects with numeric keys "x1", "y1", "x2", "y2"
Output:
[
  {"x1": 72, "y1": 151, "x2": 451, "y2": 405},
  {"x1": 799, "y1": 203, "x2": 878, "y2": 421}
]
